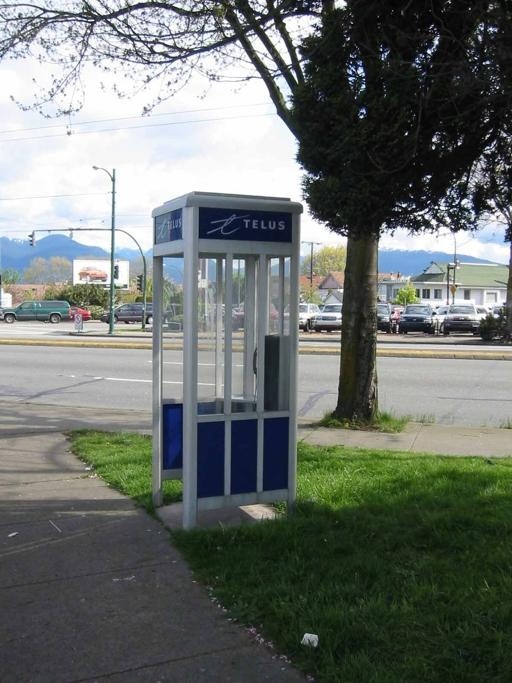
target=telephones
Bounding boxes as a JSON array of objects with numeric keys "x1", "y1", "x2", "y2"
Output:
[{"x1": 253, "y1": 335, "x2": 280, "y2": 411}]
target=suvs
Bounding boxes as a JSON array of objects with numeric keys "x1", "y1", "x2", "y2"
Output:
[
  {"x1": 307, "y1": 303, "x2": 343, "y2": 333},
  {"x1": 228, "y1": 299, "x2": 279, "y2": 331},
  {"x1": 78, "y1": 266, "x2": 109, "y2": 283},
  {"x1": 375, "y1": 303, "x2": 397, "y2": 334},
  {"x1": 274, "y1": 302, "x2": 321, "y2": 333},
  {"x1": 398, "y1": 303, "x2": 436, "y2": 335}
]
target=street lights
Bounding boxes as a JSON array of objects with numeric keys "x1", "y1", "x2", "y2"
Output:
[{"x1": 91, "y1": 164, "x2": 118, "y2": 333}]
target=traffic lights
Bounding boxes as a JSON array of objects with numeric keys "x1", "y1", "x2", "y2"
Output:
[
  {"x1": 136, "y1": 274, "x2": 144, "y2": 293},
  {"x1": 27, "y1": 233, "x2": 34, "y2": 247}
]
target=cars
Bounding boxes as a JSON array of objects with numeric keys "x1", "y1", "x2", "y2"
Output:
[
  {"x1": 69, "y1": 306, "x2": 92, "y2": 322},
  {"x1": 431, "y1": 300, "x2": 507, "y2": 336}
]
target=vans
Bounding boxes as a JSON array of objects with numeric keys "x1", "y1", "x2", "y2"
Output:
[
  {"x1": 99, "y1": 302, "x2": 168, "y2": 325},
  {"x1": 0, "y1": 300, "x2": 72, "y2": 324}
]
